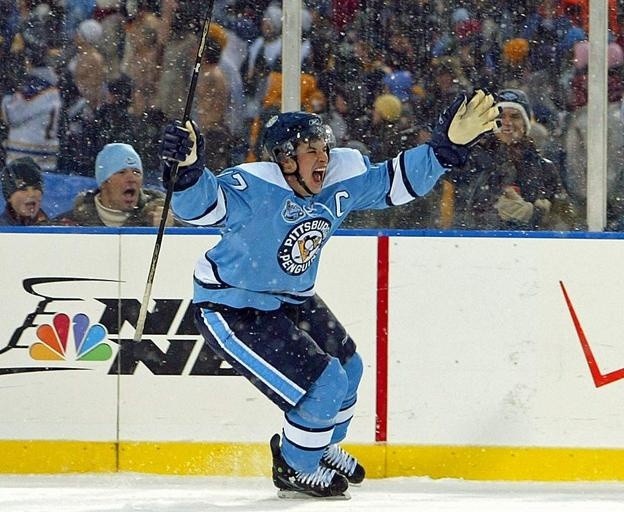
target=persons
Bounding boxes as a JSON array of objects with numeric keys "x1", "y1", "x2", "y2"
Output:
[
  {"x1": 158, "y1": 85, "x2": 501, "y2": 494},
  {"x1": 0, "y1": 0, "x2": 624, "y2": 236}
]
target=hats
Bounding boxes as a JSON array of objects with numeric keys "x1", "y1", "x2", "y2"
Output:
[
  {"x1": 497, "y1": 88, "x2": 531, "y2": 137},
  {"x1": 1, "y1": 156, "x2": 43, "y2": 201},
  {"x1": 95, "y1": 143, "x2": 144, "y2": 190}
]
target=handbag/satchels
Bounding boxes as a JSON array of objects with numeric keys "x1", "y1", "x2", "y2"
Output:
[{"x1": 425, "y1": 87, "x2": 503, "y2": 169}]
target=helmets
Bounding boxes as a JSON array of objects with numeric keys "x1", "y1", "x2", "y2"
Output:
[{"x1": 261, "y1": 111, "x2": 337, "y2": 164}]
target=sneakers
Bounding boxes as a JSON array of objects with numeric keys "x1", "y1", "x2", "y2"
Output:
[
  {"x1": 270, "y1": 433, "x2": 348, "y2": 497},
  {"x1": 319, "y1": 444, "x2": 365, "y2": 483}
]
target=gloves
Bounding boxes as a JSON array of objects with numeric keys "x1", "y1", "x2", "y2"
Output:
[{"x1": 157, "y1": 119, "x2": 206, "y2": 167}]
